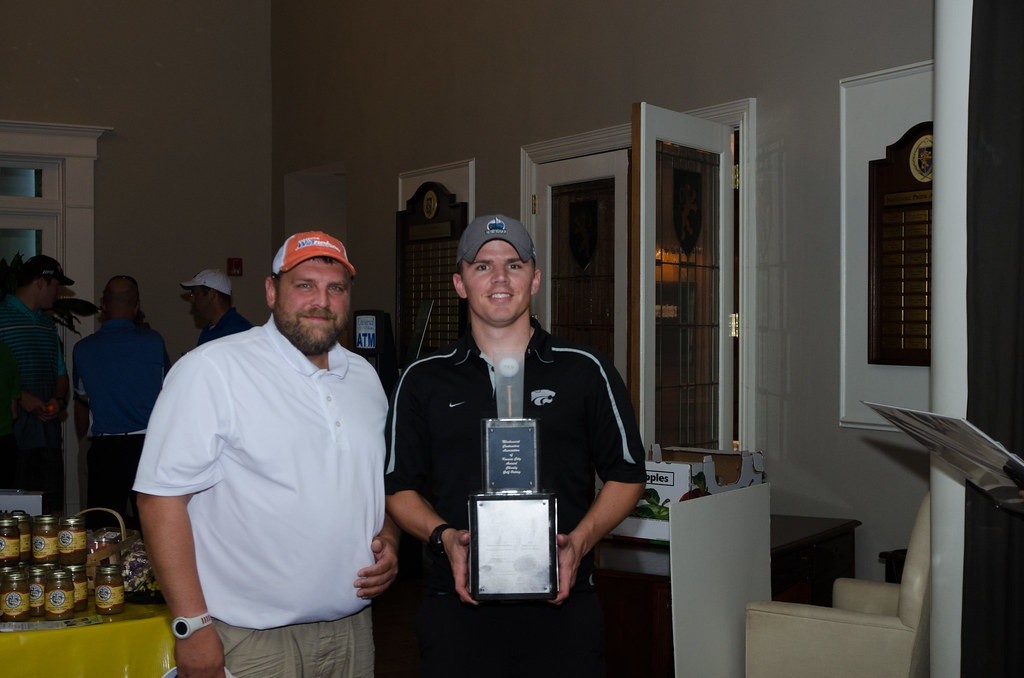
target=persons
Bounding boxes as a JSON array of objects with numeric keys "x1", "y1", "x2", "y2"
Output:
[
  {"x1": 384, "y1": 215, "x2": 647, "y2": 678},
  {"x1": 0, "y1": 339, "x2": 22, "y2": 490},
  {"x1": 132, "y1": 230, "x2": 402, "y2": 678},
  {"x1": 180, "y1": 268, "x2": 253, "y2": 347},
  {"x1": 0, "y1": 254, "x2": 76, "y2": 514},
  {"x1": 73, "y1": 275, "x2": 172, "y2": 532}
]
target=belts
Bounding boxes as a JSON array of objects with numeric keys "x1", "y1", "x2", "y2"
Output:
[{"x1": 96, "y1": 427, "x2": 149, "y2": 436}]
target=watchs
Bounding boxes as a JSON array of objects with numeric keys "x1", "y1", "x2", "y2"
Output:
[{"x1": 171, "y1": 612, "x2": 212, "y2": 639}]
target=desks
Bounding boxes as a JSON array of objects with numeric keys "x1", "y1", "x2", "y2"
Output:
[
  {"x1": 593, "y1": 515, "x2": 862, "y2": 678},
  {"x1": 0, "y1": 604, "x2": 191, "y2": 678}
]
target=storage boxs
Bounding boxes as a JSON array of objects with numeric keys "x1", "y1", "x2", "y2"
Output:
[{"x1": 594, "y1": 443, "x2": 766, "y2": 541}]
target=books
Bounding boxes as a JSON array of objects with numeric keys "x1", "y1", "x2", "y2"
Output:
[{"x1": 860, "y1": 400, "x2": 1024, "y2": 511}]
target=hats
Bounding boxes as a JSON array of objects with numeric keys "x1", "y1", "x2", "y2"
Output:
[
  {"x1": 20, "y1": 255, "x2": 75, "y2": 286},
  {"x1": 455, "y1": 215, "x2": 535, "y2": 267},
  {"x1": 271, "y1": 232, "x2": 356, "y2": 280},
  {"x1": 180, "y1": 269, "x2": 232, "y2": 298}
]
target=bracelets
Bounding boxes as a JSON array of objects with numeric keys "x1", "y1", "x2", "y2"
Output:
[
  {"x1": 428, "y1": 524, "x2": 455, "y2": 557},
  {"x1": 57, "y1": 397, "x2": 68, "y2": 410}
]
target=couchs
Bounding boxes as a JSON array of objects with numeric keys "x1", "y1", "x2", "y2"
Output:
[{"x1": 744, "y1": 489, "x2": 931, "y2": 678}]
